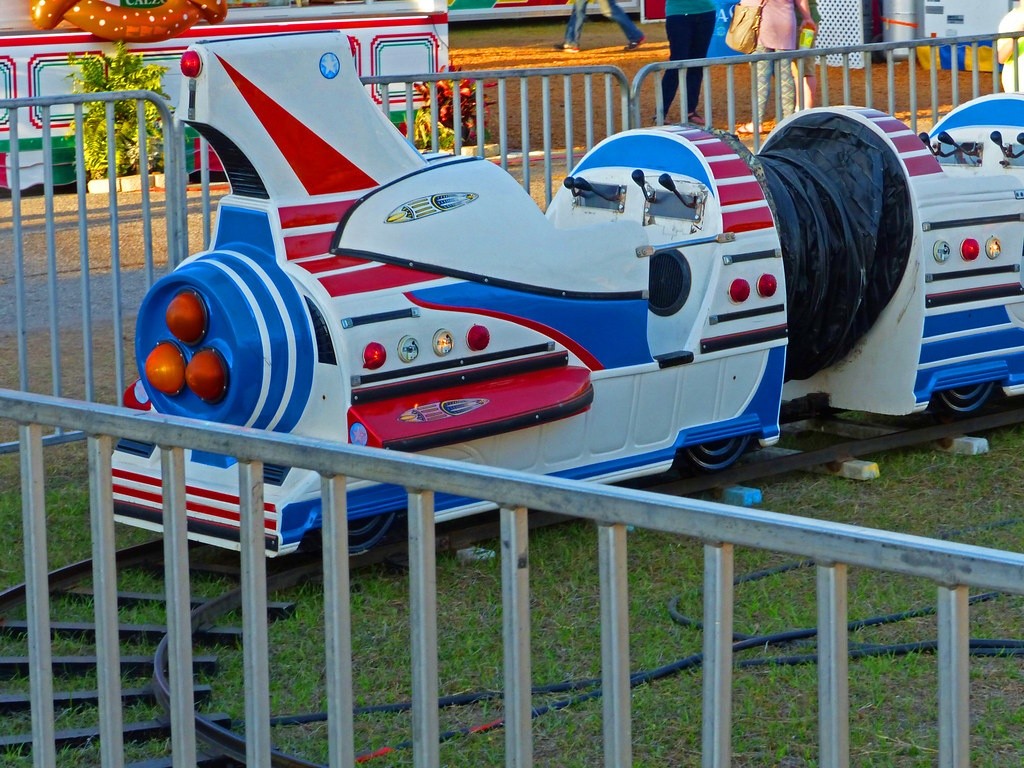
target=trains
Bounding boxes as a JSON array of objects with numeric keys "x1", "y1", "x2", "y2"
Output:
[{"x1": 112, "y1": 29, "x2": 1024, "y2": 562}]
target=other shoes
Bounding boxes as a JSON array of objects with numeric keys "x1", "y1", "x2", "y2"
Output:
[
  {"x1": 552, "y1": 43, "x2": 581, "y2": 53},
  {"x1": 623, "y1": 34, "x2": 646, "y2": 51}
]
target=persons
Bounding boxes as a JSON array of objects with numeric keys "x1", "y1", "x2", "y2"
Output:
[
  {"x1": 997, "y1": 0, "x2": 1024, "y2": 93},
  {"x1": 553, "y1": 0, "x2": 645, "y2": 53},
  {"x1": 652, "y1": 0, "x2": 716, "y2": 128},
  {"x1": 724, "y1": 0, "x2": 822, "y2": 134}
]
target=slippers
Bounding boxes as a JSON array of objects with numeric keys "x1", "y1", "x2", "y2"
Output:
[
  {"x1": 733, "y1": 122, "x2": 764, "y2": 137},
  {"x1": 653, "y1": 113, "x2": 672, "y2": 125},
  {"x1": 687, "y1": 111, "x2": 706, "y2": 124}
]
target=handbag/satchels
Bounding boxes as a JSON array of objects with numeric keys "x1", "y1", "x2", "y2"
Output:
[{"x1": 724, "y1": 3, "x2": 764, "y2": 54}]
